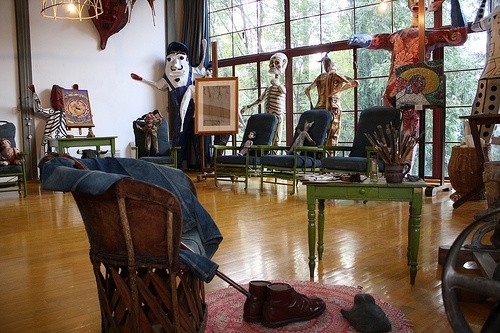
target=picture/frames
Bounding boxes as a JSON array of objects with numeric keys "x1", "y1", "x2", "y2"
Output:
[
  {"x1": 63, "y1": 90, "x2": 95, "y2": 127},
  {"x1": 194, "y1": 77, "x2": 238, "y2": 134}
]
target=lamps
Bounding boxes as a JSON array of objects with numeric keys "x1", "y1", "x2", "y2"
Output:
[{"x1": 40, "y1": 0, "x2": 104, "y2": 20}]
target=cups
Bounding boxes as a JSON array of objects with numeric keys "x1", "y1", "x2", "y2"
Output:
[{"x1": 385, "y1": 162, "x2": 411, "y2": 183}]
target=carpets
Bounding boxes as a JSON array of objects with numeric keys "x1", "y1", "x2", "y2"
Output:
[{"x1": 205, "y1": 281, "x2": 415, "y2": 333}]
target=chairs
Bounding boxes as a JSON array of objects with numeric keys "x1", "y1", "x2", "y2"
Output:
[
  {"x1": 130, "y1": 118, "x2": 181, "y2": 170},
  {"x1": 38, "y1": 157, "x2": 223, "y2": 333},
  {"x1": 209, "y1": 106, "x2": 402, "y2": 204},
  {"x1": 0, "y1": 120, "x2": 28, "y2": 198}
]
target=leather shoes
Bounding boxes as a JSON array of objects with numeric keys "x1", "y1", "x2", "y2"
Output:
[
  {"x1": 261, "y1": 282, "x2": 326, "y2": 329},
  {"x1": 243, "y1": 281, "x2": 272, "y2": 323}
]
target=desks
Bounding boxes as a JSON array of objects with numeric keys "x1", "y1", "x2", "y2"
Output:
[
  {"x1": 301, "y1": 175, "x2": 432, "y2": 285},
  {"x1": 453, "y1": 114, "x2": 500, "y2": 208},
  {"x1": 47, "y1": 136, "x2": 118, "y2": 159}
]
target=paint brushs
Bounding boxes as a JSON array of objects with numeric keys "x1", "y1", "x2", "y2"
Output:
[{"x1": 364, "y1": 122, "x2": 425, "y2": 164}]
